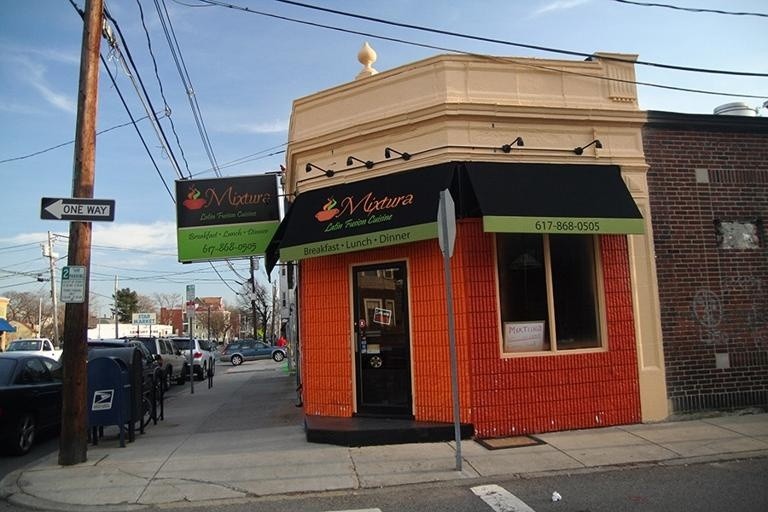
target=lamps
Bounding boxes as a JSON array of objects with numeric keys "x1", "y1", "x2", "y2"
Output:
[
  {"x1": 499, "y1": 132, "x2": 603, "y2": 157},
  {"x1": 304, "y1": 145, "x2": 411, "y2": 178}
]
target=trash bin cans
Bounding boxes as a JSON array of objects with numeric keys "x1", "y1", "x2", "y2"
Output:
[{"x1": 85, "y1": 346, "x2": 142, "y2": 425}]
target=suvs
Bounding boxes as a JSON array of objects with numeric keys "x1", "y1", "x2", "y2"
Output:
[{"x1": 219, "y1": 338, "x2": 288, "y2": 365}]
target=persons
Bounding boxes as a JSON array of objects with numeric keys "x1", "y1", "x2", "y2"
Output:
[{"x1": 277, "y1": 336, "x2": 287, "y2": 346}]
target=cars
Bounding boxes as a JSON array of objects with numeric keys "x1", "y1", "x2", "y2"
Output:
[
  {"x1": 5, "y1": 334, "x2": 218, "y2": 407},
  {"x1": 0, "y1": 350, "x2": 63, "y2": 459}
]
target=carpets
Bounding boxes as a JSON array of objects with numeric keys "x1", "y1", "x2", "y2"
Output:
[{"x1": 474, "y1": 433, "x2": 549, "y2": 451}]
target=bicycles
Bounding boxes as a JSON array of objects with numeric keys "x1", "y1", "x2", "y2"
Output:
[{"x1": 114, "y1": 371, "x2": 157, "y2": 432}]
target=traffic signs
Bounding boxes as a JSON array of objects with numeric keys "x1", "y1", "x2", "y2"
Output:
[{"x1": 38, "y1": 193, "x2": 117, "y2": 222}]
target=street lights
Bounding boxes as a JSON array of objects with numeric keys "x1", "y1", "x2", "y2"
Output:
[{"x1": 207, "y1": 304, "x2": 214, "y2": 337}]
